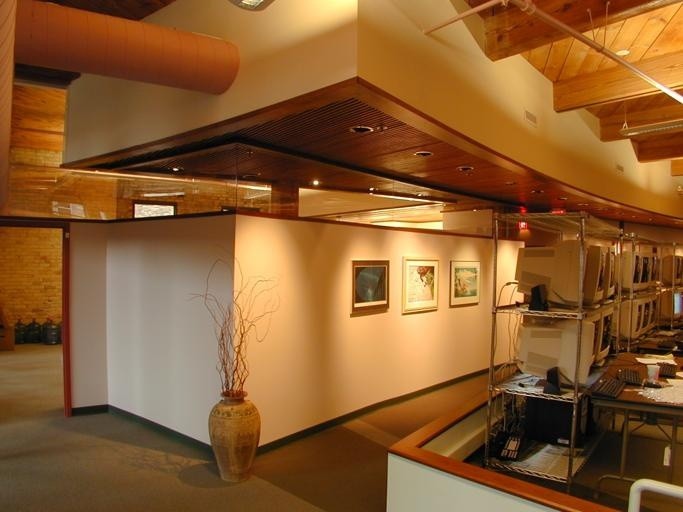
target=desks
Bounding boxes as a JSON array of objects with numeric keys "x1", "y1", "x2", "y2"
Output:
[{"x1": 586, "y1": 351, "x2": 683, "y2": 500}]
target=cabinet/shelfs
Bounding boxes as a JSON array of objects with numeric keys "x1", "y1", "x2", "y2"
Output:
[{"x1": 483, "y1": 211, "x2": 683, "y2": 495}]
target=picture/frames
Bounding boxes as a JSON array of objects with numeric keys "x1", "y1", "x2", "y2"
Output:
[{"x1": 351, "y1": 255, "x2": 481, "y2": 316}]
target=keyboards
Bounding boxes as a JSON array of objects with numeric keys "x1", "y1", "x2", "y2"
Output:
[{"x1": 591, "y1": 378, "x2": 626, "y2": 398}]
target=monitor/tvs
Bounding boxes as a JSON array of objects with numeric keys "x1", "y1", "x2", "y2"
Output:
[{"x1": 515, "y1": 241, "x2": 683, "y2": 385}]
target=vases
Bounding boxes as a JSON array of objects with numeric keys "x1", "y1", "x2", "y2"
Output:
[{"x1": 208, "y1": 389, "x2": 261, "y2": 483}]
